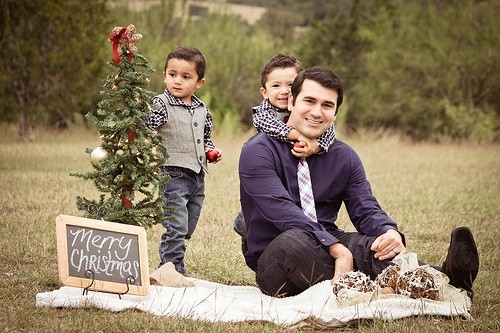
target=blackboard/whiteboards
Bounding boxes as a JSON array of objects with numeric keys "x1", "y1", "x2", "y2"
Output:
[{"x1": 55, "y1": 213, "x2": 150, "y2": 297}]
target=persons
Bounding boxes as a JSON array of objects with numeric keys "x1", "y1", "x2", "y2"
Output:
[
  {"x1": 135, "y1": 45, "x2": 223, "y2": 277},
  {"x1": 232, "y1": 51, "x2": 337, "y2": 255},
  {"x1": 238, "y1": 64, "x2": 480, "y2": 303}
]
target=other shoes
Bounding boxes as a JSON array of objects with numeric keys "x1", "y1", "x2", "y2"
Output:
[{"x1": 440, "y1": 227, "x2": 479, "y2": 301}]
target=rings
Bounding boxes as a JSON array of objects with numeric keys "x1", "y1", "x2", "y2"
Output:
[{"x1": 392, "y1": 249, "x2": 397, "y2": 255}]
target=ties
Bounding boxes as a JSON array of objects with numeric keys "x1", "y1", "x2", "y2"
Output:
[{"x1": 297, "y1": 157, "x2": 318, "y2": 223}]
